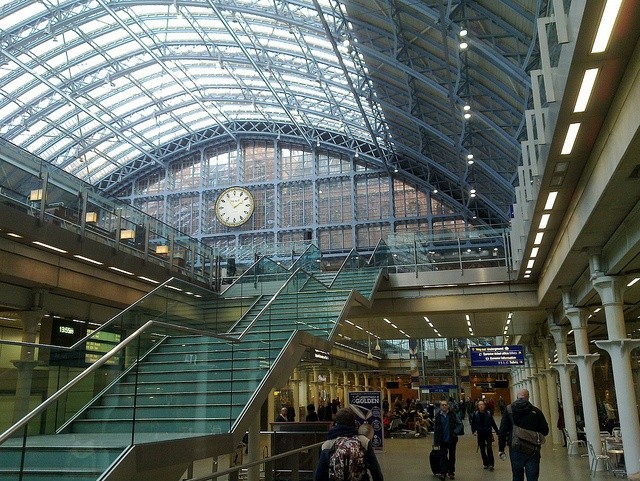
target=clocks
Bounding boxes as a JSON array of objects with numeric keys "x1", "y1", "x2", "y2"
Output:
[{"x1": 214, "y1": 187, "x2": 254, "y2": 228}]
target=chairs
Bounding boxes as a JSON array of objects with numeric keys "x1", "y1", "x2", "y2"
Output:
[
  {"x1": 563, "y1": 428, "x2": 587, "y2": 457},
  {"x1": 587, "y1": 441, "x2": 614, "y2": 479},
  {"x1": 600, "y1": 428, "x2": 623, "y2": 469}
]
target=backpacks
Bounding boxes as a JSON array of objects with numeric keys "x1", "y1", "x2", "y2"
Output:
[{"x1": 328, "y1": 434, "x2": 367, "y2": 481}]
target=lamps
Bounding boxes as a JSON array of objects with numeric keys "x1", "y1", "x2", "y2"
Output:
[
  {"x1": 368, "y1": 354, "x2": 372, "y2": 361},
  {"x1": 156, "y1": 246, "x2": 168, "y2": 254},
  {"x1": 375, "y1": 345, "x2": 381, "y2": 352},
  {"x1": 120, "y1": 230, "x2": 135, "y2": 240},
  {"x1": 29, "y1": 189, "x2": 48, "y2": 202},
  {"x1": 85, "y1": 212, "x2": 97, "y2": 223}
]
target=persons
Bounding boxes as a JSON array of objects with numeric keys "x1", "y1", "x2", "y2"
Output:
[
  {"x1": 498, "y1": 388, "x2": 550, "y2": 481},
  {"x1": 276, "y1": 408, "x2": 288, "y2": 423},
  {"x1": 557, "y1": 393, "x2": 618, "y2": 449},
  {"x1": 433, "y1": 401, "x2": 464, "y2": 481},
  {"x1": 471, "y1": 400, "x2": 502, "y2": 473},
  {"x1": 381, "y1": 395, "x2": 436, "y2": 438},
  {"x1": 497, "y1": 395, "x2": 506, "y2": 415},
  {"x1": 311, "y1": 407, "x2": 384, "y2": 481},
  {"x1": 450, "y1": 396, "x2": 477, "y2": 416},
  {"x1": 306, "y1": 404, "x2": 318, "y2": 422},
  {"x1": 318, "y1": 397, "x2": 341, "y2": 422}
]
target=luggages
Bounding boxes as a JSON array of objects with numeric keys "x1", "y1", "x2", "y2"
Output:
[{"x1": 429, "y1": 443, "x2": 450, "y2": 477}]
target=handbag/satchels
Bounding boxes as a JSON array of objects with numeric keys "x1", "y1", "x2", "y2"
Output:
[{"x1": 511, "y1": 426, "x2": 546, "y2": 452}]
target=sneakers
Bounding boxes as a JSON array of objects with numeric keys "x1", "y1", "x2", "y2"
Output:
[
  {"x1": 483, "y1": 465, "x2": 488, "y2": 471},
  {"x1": 449, "y1": 473, "x2": 455, "y2": 481},
  {"x1": 489, "y1": 466, "x2": 494, "y2": 472}
]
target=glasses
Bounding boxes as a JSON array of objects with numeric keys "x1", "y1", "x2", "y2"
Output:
[{"x1": 441, "y1": 405, "x2": 448, "y2": 408}]
